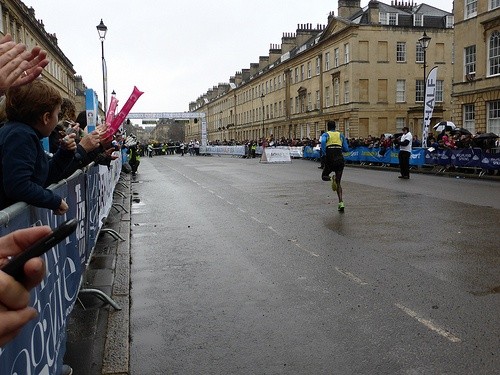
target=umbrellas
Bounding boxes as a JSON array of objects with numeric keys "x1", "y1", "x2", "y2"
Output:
[
  {"x1": 433, "y1": 121, "x2": 456, "y2": 132},
  {"x1": 449, "y1": 127, "x2": 472, "y2": 136}
]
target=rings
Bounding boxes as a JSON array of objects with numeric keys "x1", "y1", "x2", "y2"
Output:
[{"x1": 24, "y1": 71, "x2": 27, "y2": 75}]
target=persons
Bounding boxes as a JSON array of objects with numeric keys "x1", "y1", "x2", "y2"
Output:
[
  {"x1": 180, "y1": 141, "x2": 184, "y2": 156},
  {"x1": 321, "y1": 121, "x2": 349, "y2": 211},
  {"x1": 207, "y1": 139, "x2": 242, "y2": 146},
  {"x1": 300, "y1": 138, "x2": 321, "y2": 163},
  {"x1": 426, "y1": 129, "x2": 470, "y2": 174},
  {"x1": 268, "y1": 134, "x2": 302, "y2": 159},
  {"x1": 188, "y1": 140, "x2": 194, "y2": 156},
  {"x1": 396, "y1": 126, "x2": 412, "y2": 179},
  {"x1": 241, "y1": 136, "x2": 270, "y2": 159},
  {"x1": 0, "y1": 33, "x2": 140, "y2": 375},
  {"x1": 343, "y1": 134, "x2": 421, "y2": 167},
  {"x1": 175, "y1": 142, "x2": 190, "y2": 154},
  {"x1": 194, "y1": 139, "x2": 201, "y2": 156},
  {"x1": 318, "y1": 130, "x2": 326, "y2": 169},
  {"x1": 138, "y1": 142, "x2": 176, "y2": 158},
  {"x1": 468, "y1": 131, "x2": 500, "y2": 176}
]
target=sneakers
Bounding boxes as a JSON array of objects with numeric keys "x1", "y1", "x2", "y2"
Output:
[
  {"x1": 330, "y1": 175, "x2": 337, "y2": 191},
  {"x1": 337, "y1": 202, "x2": 344, "y2": 210}
]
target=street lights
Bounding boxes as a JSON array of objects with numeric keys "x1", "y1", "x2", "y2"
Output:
[
  {"x1": 418, "y1": 31, "x2": 431, "y2": 115},
  {"x1": 261, "y1": 92, "x2": 266, "y2": 136},
  {"x1": 96, "y1": 18, "x2": 107, "y2": 119}
]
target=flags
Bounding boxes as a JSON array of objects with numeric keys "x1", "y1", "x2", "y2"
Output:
[{"x1": 421, "y1": 67, "x2": 439, "y2": 148}]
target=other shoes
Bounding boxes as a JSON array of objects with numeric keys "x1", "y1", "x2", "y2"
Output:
[
  {"x1": 403, "y1": 176, "x2": 408, "y2": 179},
  {"x1": 398, "y1": 175, "x2": 403, "y2": 178}
]
